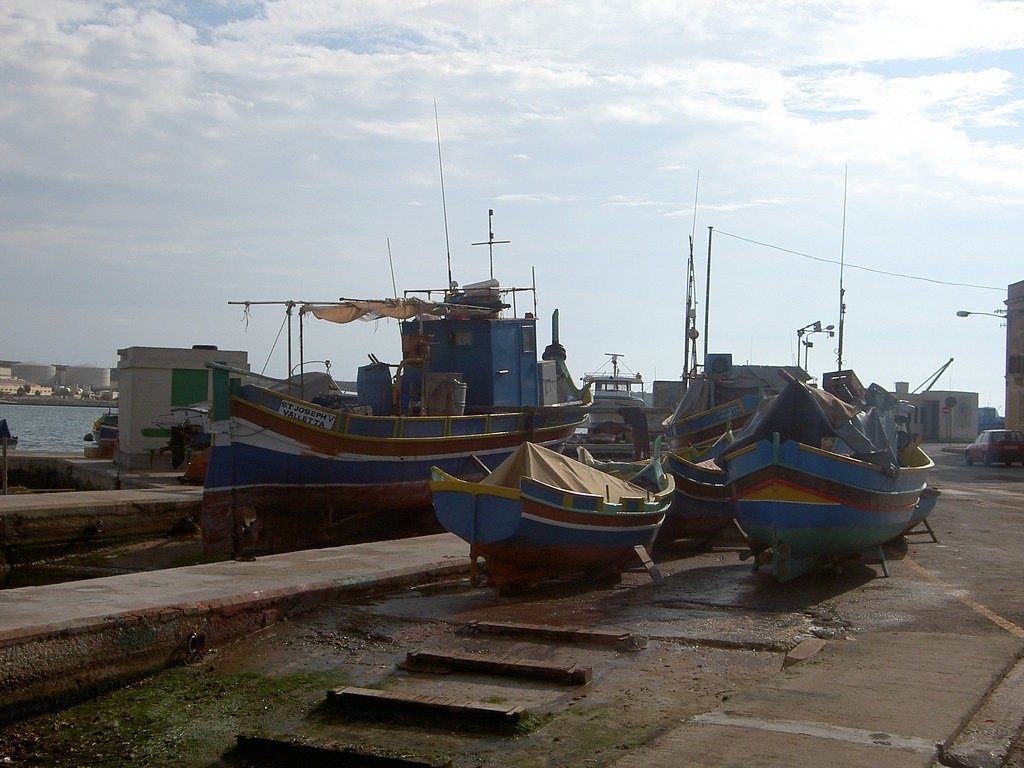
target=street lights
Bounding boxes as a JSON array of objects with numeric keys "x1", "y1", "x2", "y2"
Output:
[{"x1": 804, "y1": 324, "x2": 835, "y2": 372}]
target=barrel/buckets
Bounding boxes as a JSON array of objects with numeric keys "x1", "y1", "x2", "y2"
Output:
[
  {"x1": 437, "y1": 382, "x2": 467, "y2": 416},
  {"x1": 359, "y1": 365, "x2": 393, "y2": 416}
]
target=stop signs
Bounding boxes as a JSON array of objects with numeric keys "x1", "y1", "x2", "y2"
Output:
[{"x1": 943, "y1": 407, "x2": 950, "y2": 415}]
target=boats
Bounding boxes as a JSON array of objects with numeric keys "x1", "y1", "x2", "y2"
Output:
[{"x1": 91, "y1": 98, "x2": 944, "y2": 585}]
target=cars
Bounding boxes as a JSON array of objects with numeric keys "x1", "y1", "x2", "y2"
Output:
[{"x1": 965, "y1": 429, "x2": 1024, "y2": 468}]
